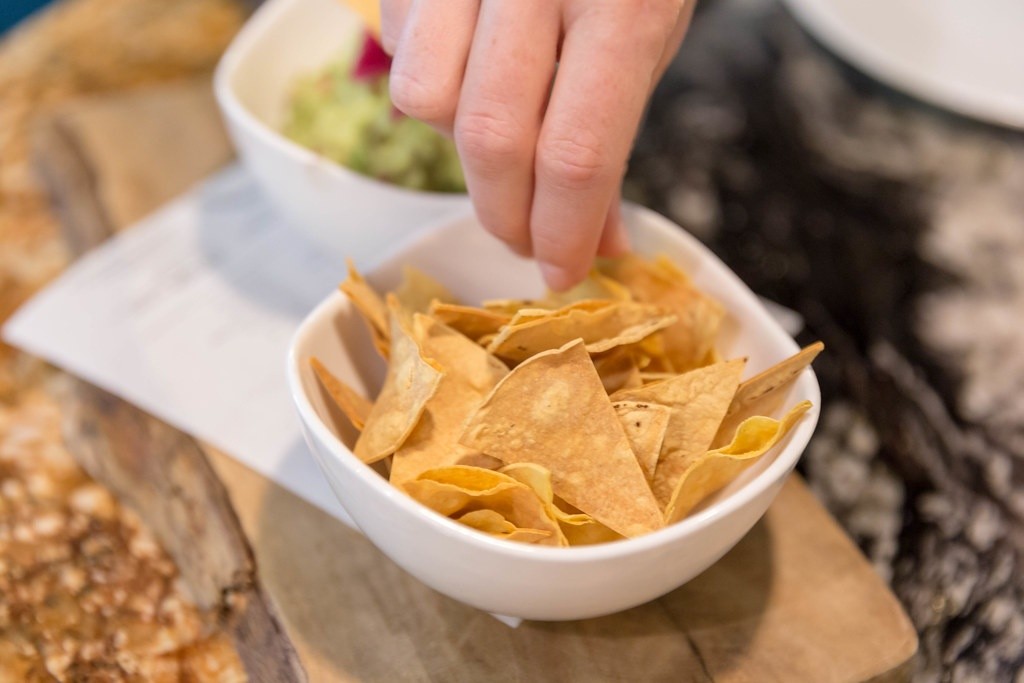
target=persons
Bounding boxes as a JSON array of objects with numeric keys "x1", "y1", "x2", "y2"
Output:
[{"x1": 380, "y1": 0, "x2": 692, "y2": 293}]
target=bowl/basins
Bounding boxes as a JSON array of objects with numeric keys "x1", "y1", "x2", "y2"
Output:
[
  {"x1": 284, "y1": 199, "x2": 824, "y2": 622},
  {"x1": 211, "y1": 0, "x2": 474, "y2": 259}
]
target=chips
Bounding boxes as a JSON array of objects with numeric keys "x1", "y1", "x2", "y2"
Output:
[{"x1": 312, "y1": 236, "x2": 825, "y2": 546}]
